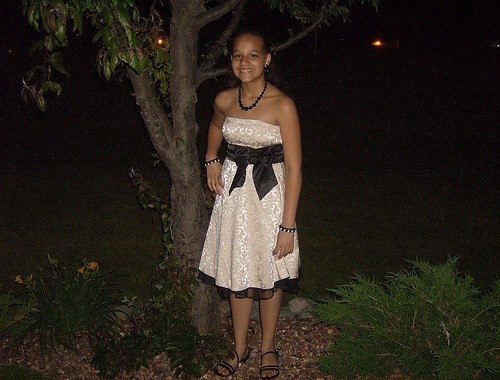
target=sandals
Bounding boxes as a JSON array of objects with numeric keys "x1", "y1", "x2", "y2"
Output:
[
  {"x1": 213, "y1": 347, "x2": 251, "y2": 377},
  {"x1": 260, "y1": 350, "x2": 280, "y2": 377}
]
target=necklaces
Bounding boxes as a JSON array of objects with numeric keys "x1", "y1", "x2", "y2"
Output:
[{"x1": 238, "y1": 81, "x2": 267, "y2": 112}]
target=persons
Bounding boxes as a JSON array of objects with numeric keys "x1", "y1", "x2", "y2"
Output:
[{"x1": 197, "y1": 27, "x2": 303, "y2": 380}]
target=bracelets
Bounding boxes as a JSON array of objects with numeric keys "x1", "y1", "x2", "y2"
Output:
[
  {"x1": 203, "y1": 157, "x2": 220, "y2": 167},
  {"x1": 278, "y1": 225, "x2": 295, "y2": 233}
]
